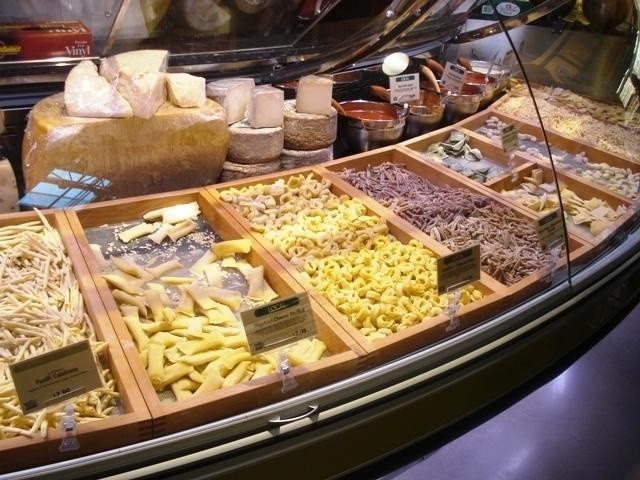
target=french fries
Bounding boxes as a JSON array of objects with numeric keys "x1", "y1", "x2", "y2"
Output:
[{"x1": 0, "y1": 202, "x2": 123, "y2": 441}]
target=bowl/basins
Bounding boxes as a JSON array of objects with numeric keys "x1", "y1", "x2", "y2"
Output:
[{"x1": 334, "y1": 58, "x2": 513, "y2": 158}]
